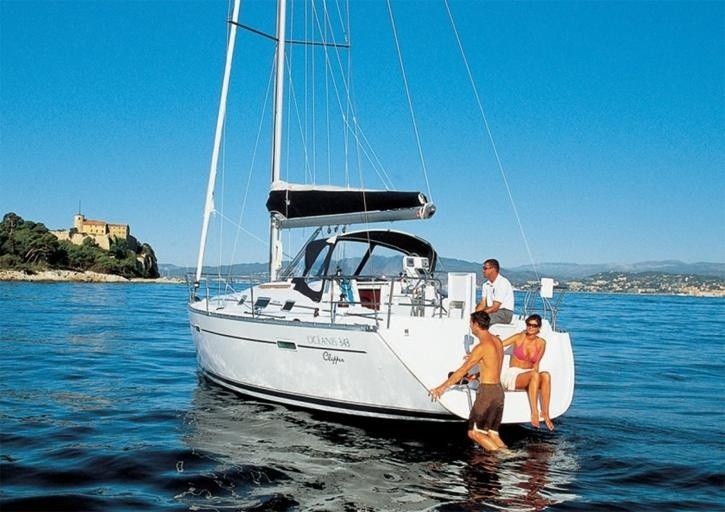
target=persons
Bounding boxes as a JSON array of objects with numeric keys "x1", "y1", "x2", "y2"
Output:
[
  {"x1": 502, "y1": 313, "x2": 556, "y2": 432},
  {"x1": 474, "y1": 258, "x2": 515, "y2": 327},
  {"x1": 518, "y1": 442, "x2": 558, "y2": 511},
  {"x1": 427, "y1": 311, "x2": 510, "y2": 452}
]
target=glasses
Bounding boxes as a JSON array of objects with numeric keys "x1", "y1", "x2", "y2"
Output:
[{"x1": 527, "y1": 323, "x2": 538, "y2": 328}]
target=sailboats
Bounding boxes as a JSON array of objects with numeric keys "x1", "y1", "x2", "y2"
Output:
[{"x1": 188, "y1": 0, "x2": 576, "y2": 423}]
